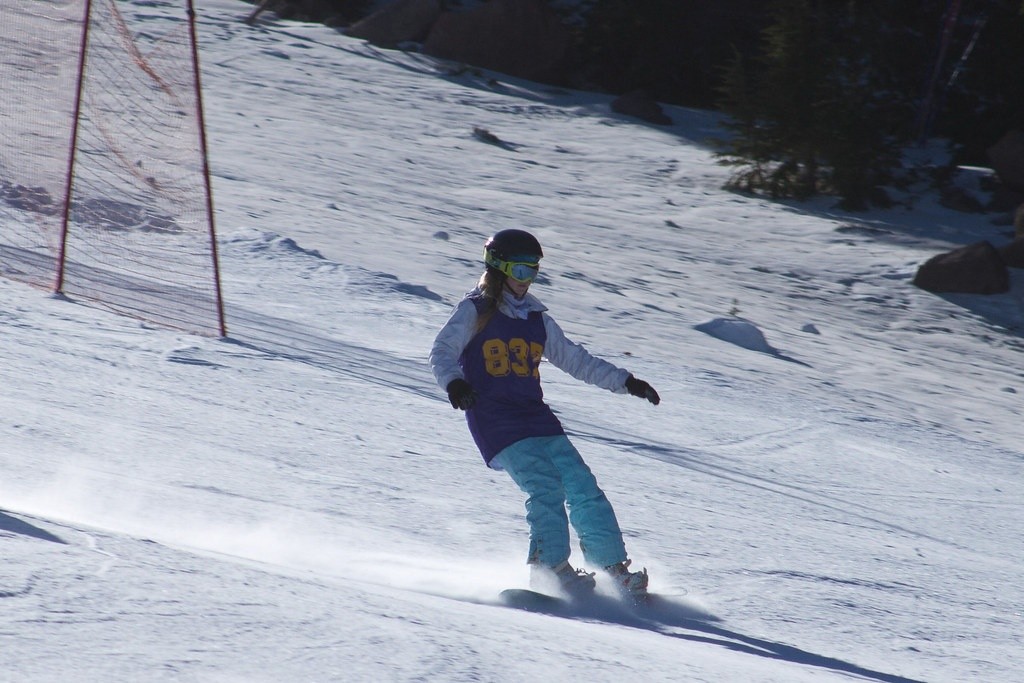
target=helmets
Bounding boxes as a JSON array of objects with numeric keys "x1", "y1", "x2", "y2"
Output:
[{"x1": 485, "y1": 229, "x2": 543, "y2": 279}]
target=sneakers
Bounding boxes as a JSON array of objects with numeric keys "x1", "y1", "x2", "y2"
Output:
[
  {"x1": 530, "y1": 560, "x2": 596, "y2": 596},
  {"x1": 607, "y1": 559, "x2": 648, "y2": 595}
]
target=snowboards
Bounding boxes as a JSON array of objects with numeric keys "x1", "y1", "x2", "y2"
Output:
[{"x1": 499, "y1": 588, "x2": 653, "y2": 618}]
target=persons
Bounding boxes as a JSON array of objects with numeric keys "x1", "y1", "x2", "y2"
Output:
[{"x1": 428, "y1": 229, "x2": 661, "y2": 610}]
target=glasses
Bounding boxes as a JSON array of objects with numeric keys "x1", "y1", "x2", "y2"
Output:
[{"x1": 512, "y1": 264, "x2": 540, "y2": 282}]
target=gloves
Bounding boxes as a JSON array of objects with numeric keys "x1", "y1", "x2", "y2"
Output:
[
  {"x1": 447, "y1": 378, "x2": 481, "y2": 410},
  {"x1": 625, "y1": 374, "x2": 660, "y2": 405}
]
